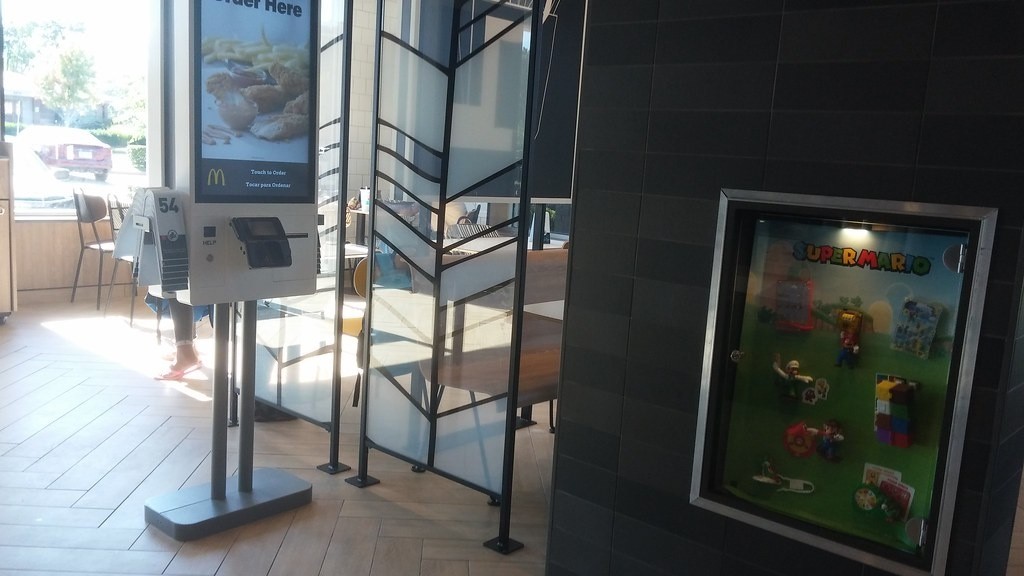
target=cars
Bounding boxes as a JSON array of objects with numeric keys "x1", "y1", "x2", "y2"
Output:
[
  {"x1": 5, "y1": 135, "x2": 76, "y2": 211},
  {"x1": 13, "y1": 126, "x2": 114, "y2": 180}
]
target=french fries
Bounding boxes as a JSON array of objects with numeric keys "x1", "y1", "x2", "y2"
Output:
[{"x1": 200, "y1": 24, "x2": 309, "y2": 70}]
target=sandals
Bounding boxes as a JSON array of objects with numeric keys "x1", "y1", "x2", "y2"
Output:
[{"x1": 153, "y1": 359, "x2": 202, "y2": 382}]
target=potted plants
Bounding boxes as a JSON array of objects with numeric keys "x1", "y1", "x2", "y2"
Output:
[{"x1": 531, "y1": 206, "x2": 554, "y2": 233}]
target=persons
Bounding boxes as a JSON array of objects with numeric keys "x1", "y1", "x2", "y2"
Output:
[
  {"x1": 772, "y1": 350, "x2": 813, "y2": 414},
  {"x1": 153, "y1": 296, "x2": 202, "y2": 381},
  {"x1": 806, "y1": 420, "x2": 845, "y2": 464},
  {"x1": 833, "y1": 326, "x2": 860, "y2": 370}
]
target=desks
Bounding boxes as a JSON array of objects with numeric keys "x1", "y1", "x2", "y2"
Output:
[
  {"x1": 257, "y1": 273, "x2": 565, "y2": 505},
  {"x1": 315, "y1": 233, "x2": 568, "y2": 293}
]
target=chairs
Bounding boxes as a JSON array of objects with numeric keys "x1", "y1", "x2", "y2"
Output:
[
  {"x1": 71, "y1": 188, "x2": 136, "y2": 312},
  {"x1": 353, "y1": 254, "x2": 412, "y2": 299}
]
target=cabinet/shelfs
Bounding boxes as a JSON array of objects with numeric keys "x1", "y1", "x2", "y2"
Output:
[
  {"x1": 349, "y1": 204, "x2": 431, "y2": 246},
  {"x1": 0, "y1": 142, "x2": 18, "y2": 323}
]
